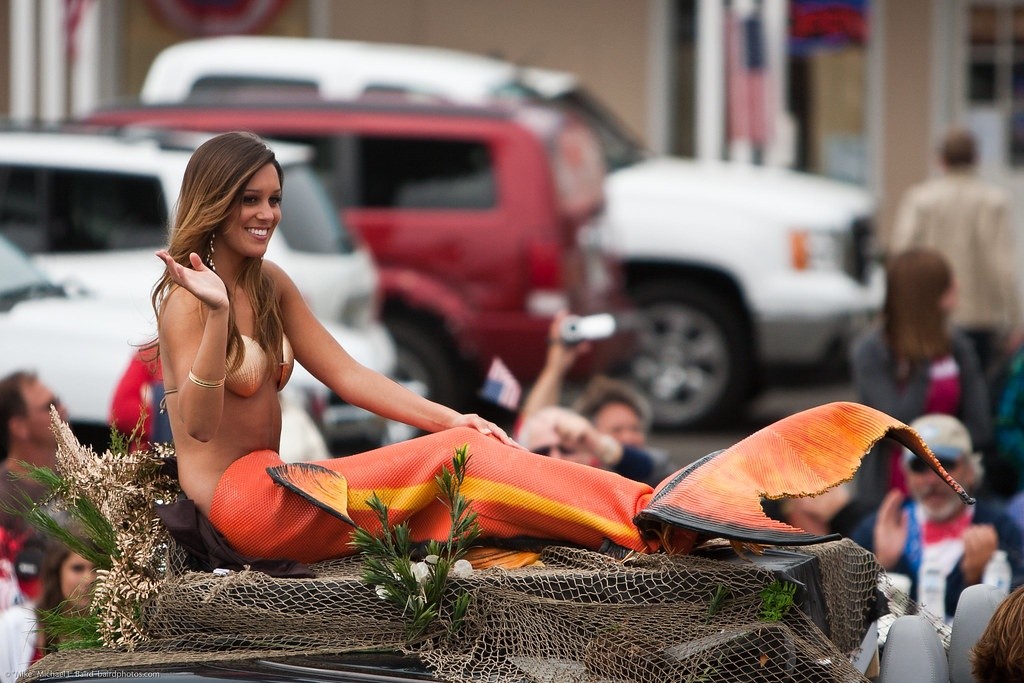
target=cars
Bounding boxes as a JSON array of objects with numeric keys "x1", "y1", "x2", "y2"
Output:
[{"x1": 2, "y1": 125, "x2": 414, "y2": 457}]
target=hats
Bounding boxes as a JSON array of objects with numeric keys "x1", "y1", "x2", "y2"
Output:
[{"x1": 903, "y1": 413, "x2": 974, "y2": 462}]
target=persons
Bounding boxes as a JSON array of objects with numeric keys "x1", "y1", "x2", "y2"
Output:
[
  {"x1": 518, "y1": 316, "x2": 680, "y2": 472},
  {"x1": 123, "y1": 128, "x2": 984, "y2": 558},
  {"x1": 515, "y1": 407, "x2": 660, "y2": 487},
  {"x1": 0, "y1": 367, "x2": 110, "y2": 683},
  {"x1": 841, "y1": 131, "x2": 1024, "y2": 683}
]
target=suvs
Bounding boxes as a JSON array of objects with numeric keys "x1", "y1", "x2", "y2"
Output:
[{"x1": 142, "y1": 37, "x2": 885, "y2": 441}]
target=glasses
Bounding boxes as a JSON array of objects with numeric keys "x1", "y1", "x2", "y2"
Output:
[{"x1": 909, "y1": 455, "x2": 962, "y2": 473}]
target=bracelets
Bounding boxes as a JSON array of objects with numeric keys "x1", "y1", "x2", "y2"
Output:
[{"x1": 187, "y1": 368, "x2": 227, "y2": 390}]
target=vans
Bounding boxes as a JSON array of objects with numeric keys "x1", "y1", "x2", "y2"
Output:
[{"x1": 59, "y1": 99, "x2": 621, "y2": 415}]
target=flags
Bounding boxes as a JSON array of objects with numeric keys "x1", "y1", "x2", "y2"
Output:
[{"x1": 481, "y1": 357, "x2": 524, "y2": 411}]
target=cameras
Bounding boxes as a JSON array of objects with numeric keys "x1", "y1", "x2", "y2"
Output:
[{"x1": 562, "y1": 309, "x2": 617, "y2": 345}]
